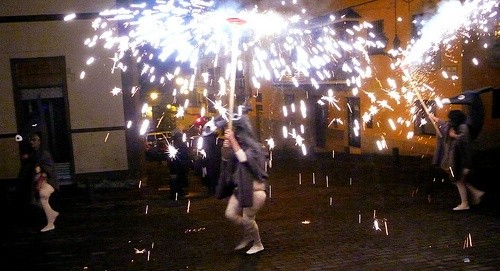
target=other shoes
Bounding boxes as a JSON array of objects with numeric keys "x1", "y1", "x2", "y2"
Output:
[
  {"x1": 474, "y1": 191, "x2": 484, "y2": 205},
  {"x1": 53, "y1": 212, "x2": 59, "y2": 223},
  {"x1": 246, "y1": 245, "x2": 264, "y2": 254},
  {"x1": 41, "y1": 225, "x2": 55, "y2": 232},
  {"x1": 453, "y1": 205, "x2": 470, "y2": 210},
  {"x1": 235, "y1": 239, "x2": 254, "y2": 250}
]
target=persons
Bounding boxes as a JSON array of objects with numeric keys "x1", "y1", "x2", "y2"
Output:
[
  {"x1": 164, "y1": 113, "x2": 269, "y2": 255},
  {"x1": 21, "y1": 133, "x2": 60, "y2": 232},
  {"x1": 431, "y1": 110, "x2": 484, "y2": 211}
]
